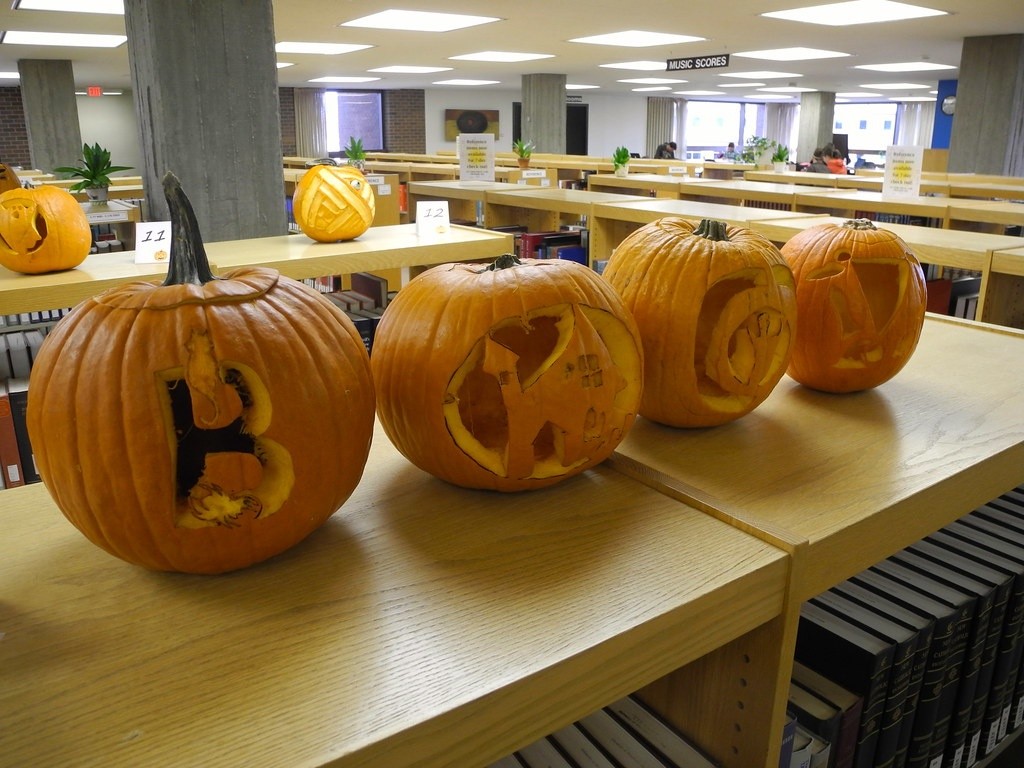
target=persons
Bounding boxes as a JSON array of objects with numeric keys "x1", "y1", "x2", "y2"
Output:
[
  {"x1": 806, "y1": 142, "x2": 847, "y2": 174},
  {"x1": 654, "y1": 142, "x2": 677, "y2": 159},
  {"x1": 724, "y1": 142, "x2": 738, "y2": 159}
]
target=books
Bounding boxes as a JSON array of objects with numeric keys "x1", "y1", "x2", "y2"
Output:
[
  {"x1": 0, "y1": 307, "x2": 74, "y2": 494},
  {"x1": 777, "y1": 481, "x2": 1024, "y2": 768},
  {"x1": 286, "y1": 195, "x2": 302, "y2": 232},
  {"x1": 89, "y1": 199, "x2": 149, "y2": 255},
  {"x1": 744, "y1": 198, "x2": 792, "y2": 212},
  {"x1": 299, "y1": 271, "x2": 399, "y2": 359},
  {"x1": 492, "y1": 692, "x2": 723, "y2": 768},
  {"x1": 854, "y1": 210, "x2": 943, "y2": 229},
  {"x1": 917, "y1": 261, "x2": 982, "y2": 321},
  {"x1": 476, "y1": 179, "x2": 608, "y2": 274}
]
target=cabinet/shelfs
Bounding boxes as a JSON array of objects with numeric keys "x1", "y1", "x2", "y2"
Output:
[{"x1": 1, "y1": 151, "x2": 1024, "y2": 768}]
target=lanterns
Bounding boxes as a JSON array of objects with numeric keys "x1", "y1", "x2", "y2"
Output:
[
  {"x1": 368, "y1": 251, "x2": 644, "y2": 491},
  {"x1": 26, "y1": 266, "x2": 376, "y2": 576},
  {"x1": 779, "y1": 221, "x2": 926, "y2": 395},
  {"x1": 601, "y1": 216, "x2": 799, "y2": 430},
  {"x1": 0, "y1": 185, "x2": 92, "y2": 274}
]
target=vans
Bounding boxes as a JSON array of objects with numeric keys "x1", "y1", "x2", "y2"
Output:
[{"x1": 685, "y1": 150, "x2": 715, "y2": 177}]
